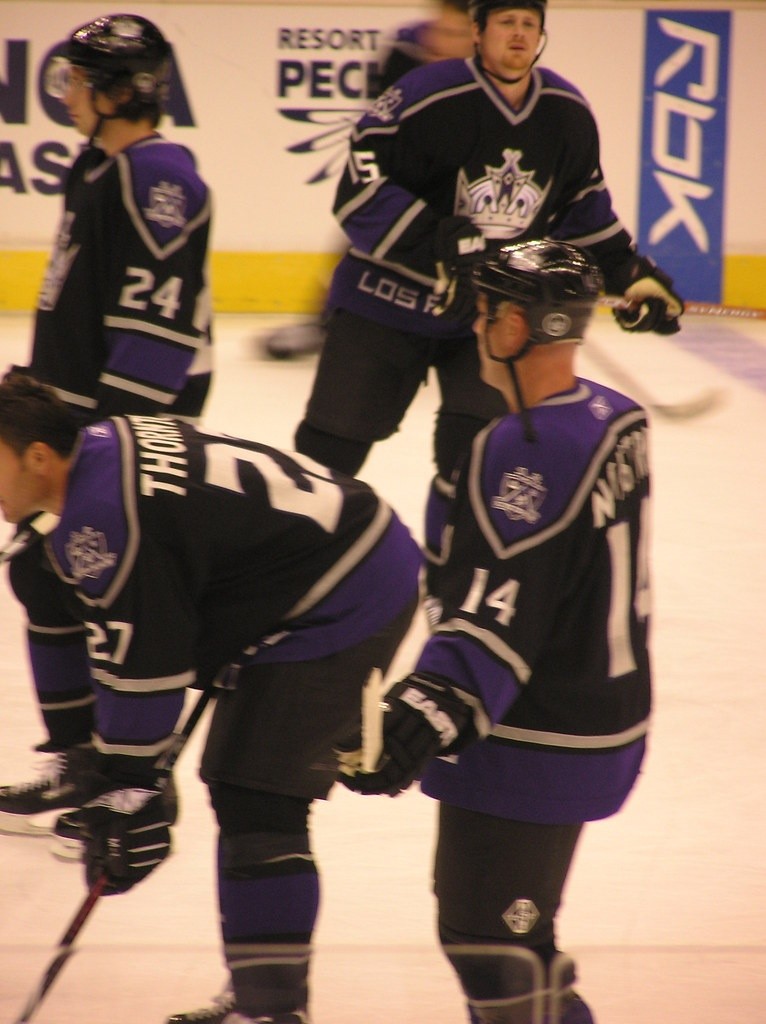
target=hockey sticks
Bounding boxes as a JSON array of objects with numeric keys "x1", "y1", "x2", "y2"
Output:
[
  {"x1": 577, "y1": 337, "x2": 726, "y2": 424},
  {"x1": 11, "y1": 686, "x2": 214, "y2": 1024}
]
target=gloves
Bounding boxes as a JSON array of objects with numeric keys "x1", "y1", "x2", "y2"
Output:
[
  {"x1": 432, "y1": 216, "x2": 484, "y2": 288},
  {"x1": 333, "y1": 671, "x2": 473, "y2": 796},
  {"x1": 611, "y1": 269, "x2": 684, "y2": 335},
  {"x1": 85, "y1": 781, "x2": 174, "y2": 896}
]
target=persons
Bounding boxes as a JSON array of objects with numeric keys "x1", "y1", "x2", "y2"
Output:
[
  {"x1": 0, "y1": 375, "x2": 435, "y2": 1023},
  {"x1": 306, "y1": 240, "x2": 654, "y2": 1023},
  {"x1": 1, "y1": 13, "x2": 214, "y2": 862},
  {"x1": 246, "y1": 1, "x2": 688, "y2": 478}
]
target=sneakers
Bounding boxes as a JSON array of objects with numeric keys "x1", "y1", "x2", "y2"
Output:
[
  {"x1": 51, "y1": 775, "x2": 179, "y2": 864},
  {"x1": 166, "y1": 993, "x2": 306, "y2": 1024},
  {"x1": 0, "y1": 737, "x2": 96, "y2": 839}
]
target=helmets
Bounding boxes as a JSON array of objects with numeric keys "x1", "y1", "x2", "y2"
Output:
[
  {"x1": 468, "y1": 0, "x2": 549, "y2": 34},
  {"x1": 62, "y1": 15, "x2": 169, "y2": 119},
  {"x1": 471, "y1": 239, "x2": 597, "y2": 346}
]
